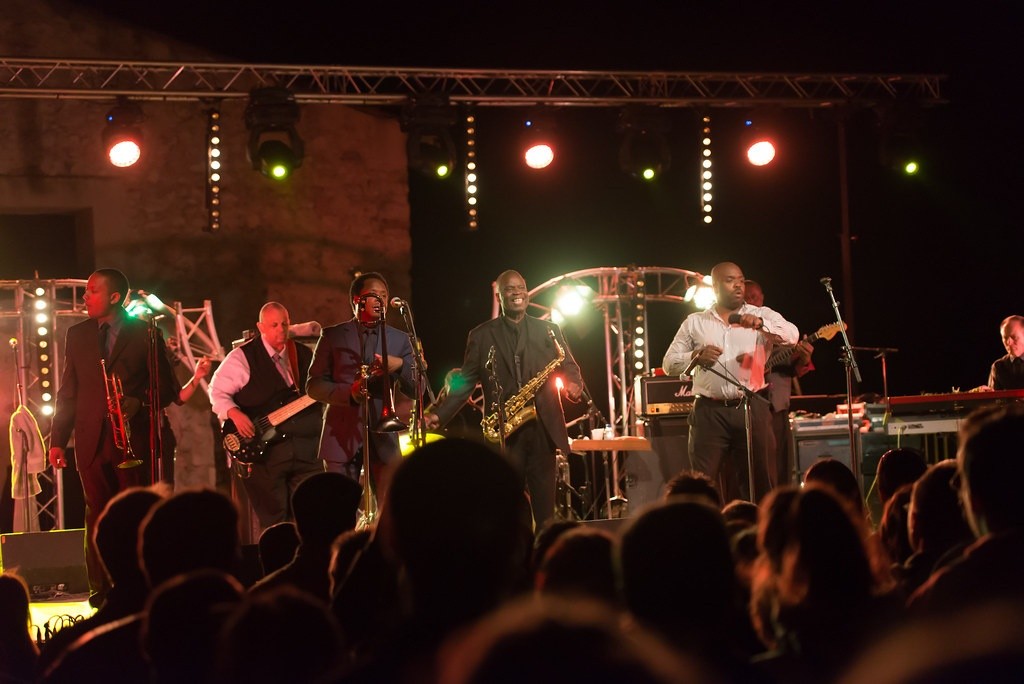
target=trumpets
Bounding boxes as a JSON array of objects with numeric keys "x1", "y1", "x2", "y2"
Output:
[{"x1": 100, "y1": 357, "x2": 145, "y2": 469}]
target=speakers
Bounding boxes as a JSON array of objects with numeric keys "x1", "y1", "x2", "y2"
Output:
[
  {"x1": 0, "y1": 528, "x2": 90, "y2": 593},
  {"x1": 636, "y1": 415, "x2": 726, "y2": 504}
]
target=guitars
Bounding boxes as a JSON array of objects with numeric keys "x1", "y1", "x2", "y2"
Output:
[
  {"x1": 221, "y1": 393, "x2": 317, "y2": 466},
  {"x1": 765, "y1": 320, "x2": 848, "y2": 372}
]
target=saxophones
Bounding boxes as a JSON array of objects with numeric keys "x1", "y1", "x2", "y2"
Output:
[{"x1": 481, "y1": 325, "x2": 566, "y2": 443}]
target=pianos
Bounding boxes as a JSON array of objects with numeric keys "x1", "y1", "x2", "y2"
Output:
[{"x1": 885, "y1": 388, "x2": 1024, "y2": 436}]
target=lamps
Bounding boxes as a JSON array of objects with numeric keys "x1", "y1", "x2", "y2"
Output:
[
  {"x1": 244, "y1": 84, "x2": 303, "y2": 180},
  {"x1": 515, "y1": 118, "x2": 553, "y2": 170},
  {"x1": 742, "y1": 121, "x2": 777, "y2": 168},
  {"x1": 100, "y1": 98, "x2": 144, "y2": 169}
]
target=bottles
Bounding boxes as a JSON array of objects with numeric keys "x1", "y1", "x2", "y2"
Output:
[{"x1": 604, "y1": 424, "x2": 614, "y2": 441}]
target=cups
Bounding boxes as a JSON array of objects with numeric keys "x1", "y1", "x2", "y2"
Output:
[{"x1": 591, "y1": 427, "x2": 603, "y2": 440}]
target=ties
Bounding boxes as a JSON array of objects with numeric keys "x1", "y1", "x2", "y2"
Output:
[
  {"x1": 272, "y1": 354, "x2": 291, "y2": 387},
  {"x1": 364, "y1": 329, "x2": 374, "y2": 364},
  {"x1": 100, "y1": 323, "x2": 110, "y2": 360},
  {"x1": 515, "y1": 325, "x2": 530, "y2": 385}
]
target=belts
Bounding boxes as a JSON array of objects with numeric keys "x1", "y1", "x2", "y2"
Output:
[{"x1": 696, "y1": 398, "x2": 744, "y2": 407}]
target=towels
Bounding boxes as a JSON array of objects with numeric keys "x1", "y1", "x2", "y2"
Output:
[{"x1": 9, "y1": 406, "x2": 44, "y2": 501}]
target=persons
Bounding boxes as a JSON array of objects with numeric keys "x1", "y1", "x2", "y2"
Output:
[
  {"x1": 744, "y1": 280, "x2": 814, "y2": 503},
  {"x1": 0, "y1": 398, "x2": 1024, "y2": 684},
  {"x1": 662, "y1": 262, "x2": 799, "y2": 504},
  {"x1": 48, "y1": 268, "x2": 174, "y2": 608},
  {"x1": 988, "y1": 315, "x2": 1024, "y2": 390},
  {"x1": 207, "y1": 302, "x2": 325, "y2": 529},
  {"x1": 305, "y1": 272, "x2": 427, "y2": 510},
  {"x1": 158, "y1": 328, "x2": 212, "y2": 484},
  {"x1": 417, "y1": 270, "x2": 584, "y2": 534}
]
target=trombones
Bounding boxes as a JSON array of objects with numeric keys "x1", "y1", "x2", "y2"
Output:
[{"x1": 356, "y1": 292, "x2": 404, "y2": 528}]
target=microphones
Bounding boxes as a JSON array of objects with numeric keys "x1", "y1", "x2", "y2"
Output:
[
  {"x1": 390, "y1": 297, "x2": 407, "y2": 308},
  {"x1": 485, "y1": 346, "x2": 497, "y2": 371},
  {"x1": 680, "y1": 349, "x2": 704, "y2": 382},
  {"x1": 728, "y1": 313, "x2": 763, "y2": 326}
]
